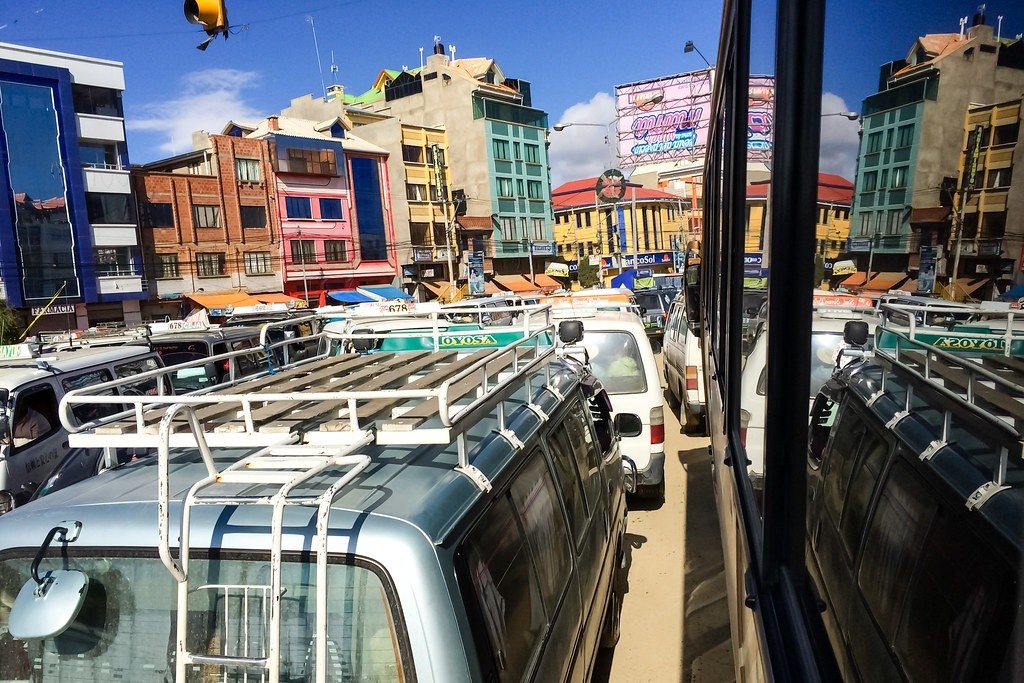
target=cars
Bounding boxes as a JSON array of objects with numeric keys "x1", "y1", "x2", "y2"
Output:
[{"x1": 0, "y1": 267, "x2": 1023, "y2": 683}]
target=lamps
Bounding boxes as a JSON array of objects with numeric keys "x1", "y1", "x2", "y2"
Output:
[{"x1": 683, "y1": 40, "x2": 713, "y2": 67}]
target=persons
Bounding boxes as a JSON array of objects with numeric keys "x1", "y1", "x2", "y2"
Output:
[{"x1": 7, "y1": 391, "x2": 51, "y2": 447}]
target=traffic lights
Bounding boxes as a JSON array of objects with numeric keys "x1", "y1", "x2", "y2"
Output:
[{"x1": 184, "y1": 0, "x2": 230, "y2": 36}]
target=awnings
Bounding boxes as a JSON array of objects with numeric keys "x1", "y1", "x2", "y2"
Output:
[
  {"x1": 860, "y1": 273, "x2": 909, "y2": 293},
  {"x1": 188, "y1": 291, "x2": 264, "y2": 310},
  {"x1": 421, "y1": 280, "x2": 504, "y2": 299},
  {"x1": 492, "y1": 274, "x2": 539, "y2": 296},
  {"x1": 326, "y1": 289, "x2": 377, "y2": 303},
  {"x1": 841, "y1": 271, "x2": 878, "y2": 291},
  {"x1": 356, "y1": 284, "x2": 415, "y2": 302},
  {"x1": 1001, "y1": 284, "x2": 1024, "y2": 301},
  {"x1": 897, "y1": 278, "x2": 990, "y2": 300},
  {"x1": 249, "y1": 293, "x2": 308, "y2": 309},
  {"x1": 523, "y1": 274, "x2": 562, "y2": 291}
]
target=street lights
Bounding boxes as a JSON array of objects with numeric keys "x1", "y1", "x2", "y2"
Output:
[
  {"x1": 552, "y1": 93, "x2": 665, "y2": 276},
  {"x1": 305, "y1": 15, "x2": 329, "y2": 104}
]
